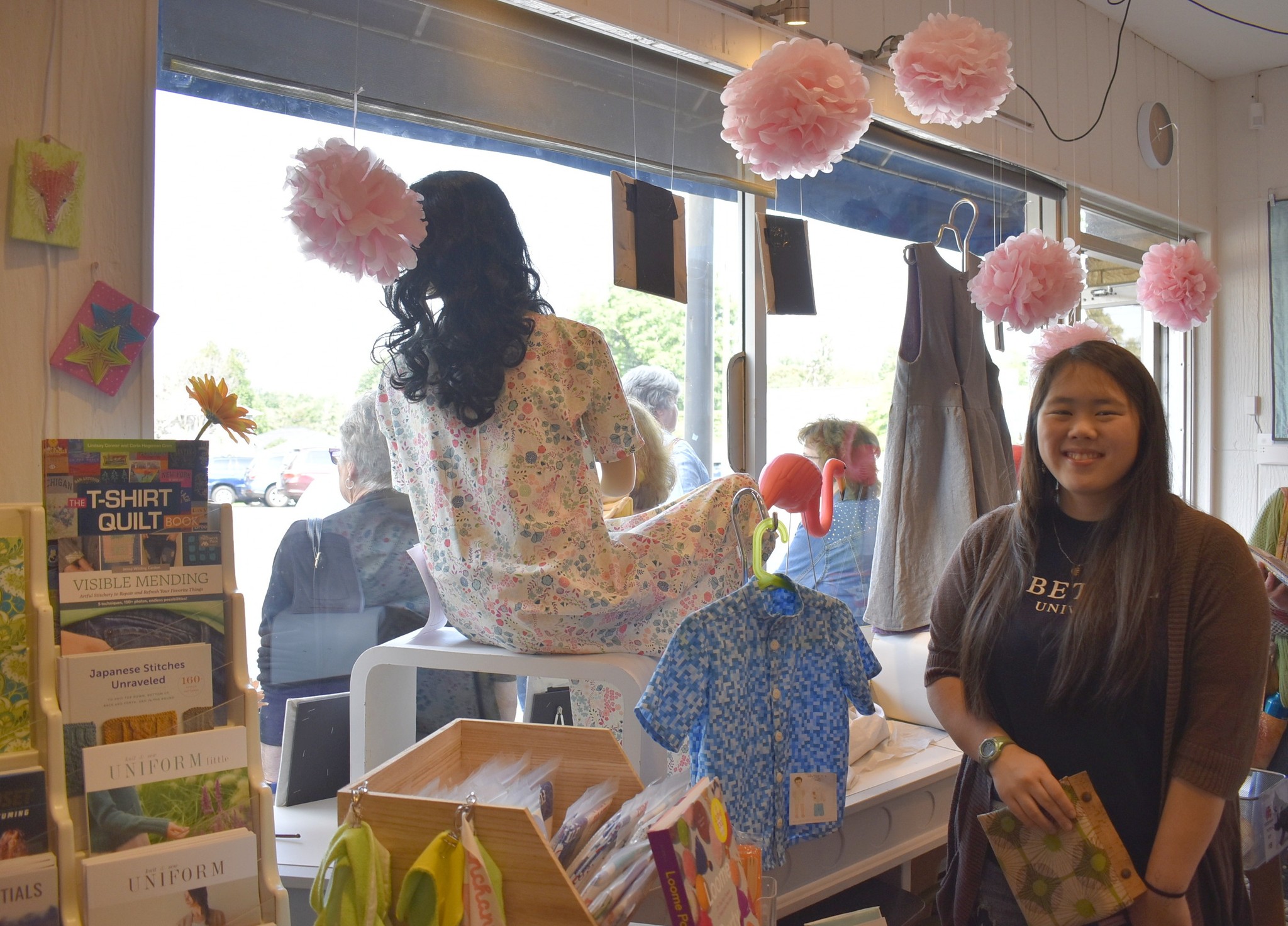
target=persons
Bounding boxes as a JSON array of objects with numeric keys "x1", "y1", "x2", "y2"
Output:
[
  {"x1": 773, "y1": 417, "x2": 882, "y2": 631},
  {"x1": 599, "y1": 396, "x2": 677, "y2": 518},
  {"x1": 923, "y1": 339, "x2": 1273, "y2": 926},
  {"x1": 257, "y1": 389, "x2": 519, "y2": 792},
  {"x1": 374, "y1": 167, "x2": 776, "y2": 653},
  {"x1": 617, "y1": 364, "x2": 709, "y2": 494},
  {"x1": 1247, "y1": 486, "x2": 1288, "y2": 925}
]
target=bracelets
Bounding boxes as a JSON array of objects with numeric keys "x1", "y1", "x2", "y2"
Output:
[{"x1": 266, "y1": 780, "x2": 278, "y2": 794}]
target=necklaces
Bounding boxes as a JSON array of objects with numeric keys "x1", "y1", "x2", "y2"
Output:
[{"x1": 1052, "y1": 495, "x2": 1091, "y2": 576}]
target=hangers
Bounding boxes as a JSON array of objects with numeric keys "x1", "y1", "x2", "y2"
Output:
[{"x1": 902, "y1": 196, "x2": 979, "y2": 272}]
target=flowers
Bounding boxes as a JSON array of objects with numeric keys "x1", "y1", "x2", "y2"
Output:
[
  {"x1": 967, "y1": 228, "x2": 1087, "y2": 334},
  {"x1": 279, "y1": 136, "x2": 427, "y2": 283},
  {"x1": 184, "y1": 374, "x2": 258, "y2": 445},
  {"x1": 887, "y1": 11, "x2": 1018, "y2": 127},
  {"x1": 720, "y1": 38, "x2": 874, "y2": 180},
  {"x1": 1135, "y1": 238, "x2": 1220, "y2": 333},
  {"x1": 1024, "y1": 318, "x2": 1116, "y2": 384}
]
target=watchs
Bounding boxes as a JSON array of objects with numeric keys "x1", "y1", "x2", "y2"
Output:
[{"x1": 979, "y1": 735, "x2": 1014, "y2": 777}]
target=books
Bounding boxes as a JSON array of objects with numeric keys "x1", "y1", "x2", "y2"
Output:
[
  {"x1": 647, "y1": 775, "x2": 759, "y2": 926},
  {"x1": 1, "y1": 436, "x2": 263, "y2": 926}
]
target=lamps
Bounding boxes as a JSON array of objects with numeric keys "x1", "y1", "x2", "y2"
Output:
[{"x1": 1137, "y1": 101, "x2": 1174, "y2": 169}]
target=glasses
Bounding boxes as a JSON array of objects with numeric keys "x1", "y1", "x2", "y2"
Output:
[
  {"x1": 329, "y1": 448, "x2": 345, "y2": 465},
  {"x1": 662, "y1": 397, "x2": 684, "y2": 411},
  {"x1": 803, "y1": 453, "x2": 821, "y2": 462}
]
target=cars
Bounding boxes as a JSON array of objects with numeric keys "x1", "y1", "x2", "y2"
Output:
[
  {"x1": 283, "y1": 448, "x2": 331, "y2": 505},
  {"x1": 207, "y1": 455, "x2": 259, "y2": 504},
  {"x1": 244, "y1": 457, "x2": 293, "y2": 507}
]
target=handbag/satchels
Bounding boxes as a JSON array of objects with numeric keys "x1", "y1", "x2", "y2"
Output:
[{"x1": 261, "y1": 688, "x2": 320, "y2": 746}]
